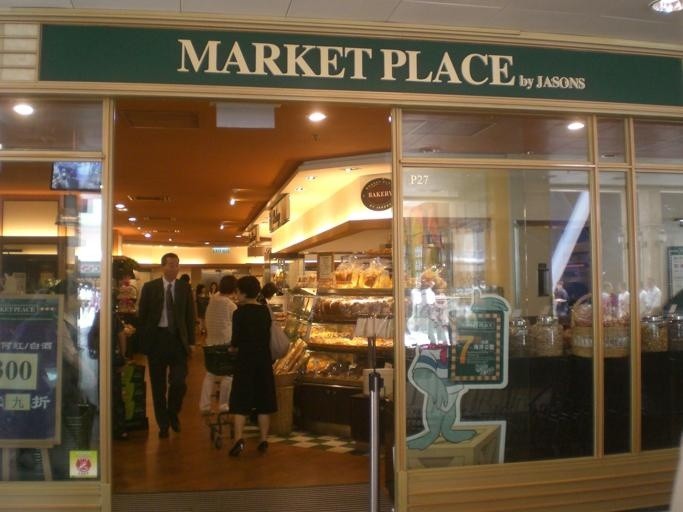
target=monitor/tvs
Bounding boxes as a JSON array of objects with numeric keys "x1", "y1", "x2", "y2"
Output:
[{"x1": 49, "y1": 161, "x2": 103, "y2": 192}]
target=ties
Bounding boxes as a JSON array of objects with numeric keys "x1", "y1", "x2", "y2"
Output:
[{"x1": 165, "y1": 284, "x2": 176, "y2": 328}]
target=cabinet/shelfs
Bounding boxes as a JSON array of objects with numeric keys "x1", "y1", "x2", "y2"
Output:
[{"x1": 286, "y1": 286, "x2": 507, "y2": 437}]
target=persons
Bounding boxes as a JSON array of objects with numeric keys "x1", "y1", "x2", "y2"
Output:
[
  {"x1": 196, "y1": 284, "x2": 208, "y2": 334},
  {"x1": 228, "y1": 275, "x2": 277, "y2": 457},
  {"x1": 209, "y1": 282, "x2": 220, "y2": 299},
  {"x1": 110, "y1": 310, "x2": 128, "y2": 438},
  {"x1": 47, "y1": 281, "x2": 97, "y2": 481},
  {"x1": 409, "y1": 270, "x2": 439, "y2": 344},
  {"x1": 114, "y1": 284, "x2": 147, "y2": 432},
  {"x1": 197, "y1": 275, "x2": 238, "y2": 415},
  {"x1": 255, "y1": 281, "x2": 278, "y2": 305},
  {"x1": 136, "y1": 253, "x2": 197, "y2": 437},
  {"x1": 552, "y1": 276, "x2": 662, "y2": 318},
  {"x1": 431, "y1": 277, "x2": 451, "y2": 347}
]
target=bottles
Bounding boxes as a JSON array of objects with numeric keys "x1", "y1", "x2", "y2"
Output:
[{"x1": 508, "y1": 307, "x2": 683, "y2": 359}]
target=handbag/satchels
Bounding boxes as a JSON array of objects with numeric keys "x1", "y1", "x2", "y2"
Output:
[{"x1": 266, "y1": 304, "x2": 291, "y2": 360}]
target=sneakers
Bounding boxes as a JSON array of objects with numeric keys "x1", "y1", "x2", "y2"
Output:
[
  {"x1": 170, "y1": 411, "x2": 181, "y2": 433},
  {"x1": 202, "y1": 408, "x2": 216, "y2": 416},
  {"x1": 159, "y1": 426, "x2": 170, "y2": 439}
]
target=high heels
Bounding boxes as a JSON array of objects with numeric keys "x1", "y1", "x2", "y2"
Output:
[
  {"x1": 257, "y1": 440, "x2": 269, "y2": 455},
  {"x1": 229, "y1": 438, "x2": 245, "y2": 456}
]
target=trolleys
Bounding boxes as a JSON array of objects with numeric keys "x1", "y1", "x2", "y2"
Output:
[{"x1": 200, "y1": 343, "x2": 237, "y2": 450}]
